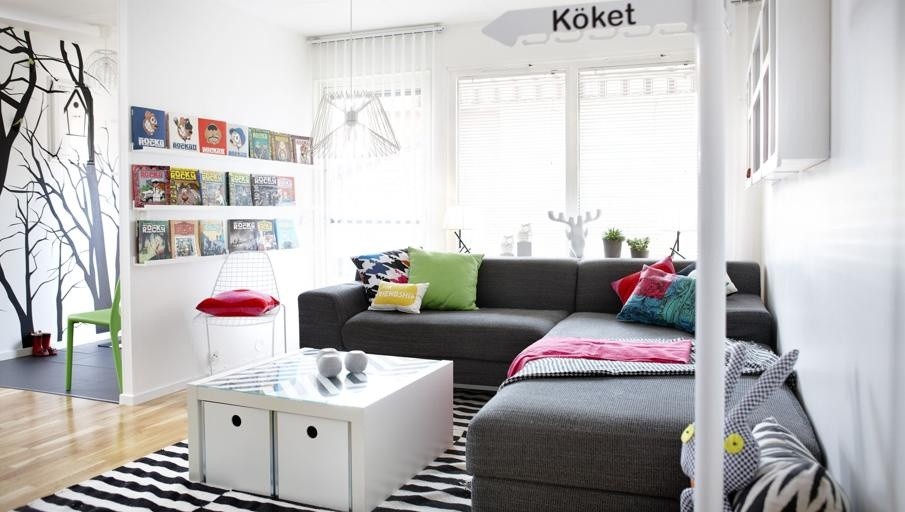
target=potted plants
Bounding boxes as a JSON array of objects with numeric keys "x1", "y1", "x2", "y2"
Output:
[
  {"x1": 627, "y1": 236, "x2": 649, "y2": 257},
  {"x1": 602, "y1": 227, "x2": 626, "y2": 257}
]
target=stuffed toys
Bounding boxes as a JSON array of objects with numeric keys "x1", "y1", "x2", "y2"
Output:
[{"x1": 680, "y1": 341, "x2": 799, "y2": 512}]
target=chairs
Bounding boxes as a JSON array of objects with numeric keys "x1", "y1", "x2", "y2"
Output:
[
  {"x1": 198, "y1": 250, "x2": 287, "y2": 375},
  {"x1": 65, "y1": 278, "x2": 122, "y2": 395}
]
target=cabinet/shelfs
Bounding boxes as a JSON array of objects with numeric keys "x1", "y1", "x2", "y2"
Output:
[
  {"x1": 744, "y1": 1, "x2": 831, "y2": 190},
  {"x1": 128, "y1": 142, "x2": 319, "y2": 267}
]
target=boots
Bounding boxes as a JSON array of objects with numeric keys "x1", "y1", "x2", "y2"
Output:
[
  {"x1": 38, "y1": 331, "x2": 58, "y2": 355},
  {"x1": 30, "y1": 332, "x2": 51, "y2": 356}
]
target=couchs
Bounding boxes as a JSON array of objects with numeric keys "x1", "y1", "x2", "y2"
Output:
[{"x1": 298, "y1": 257, "x2": 826, "y2": 511}]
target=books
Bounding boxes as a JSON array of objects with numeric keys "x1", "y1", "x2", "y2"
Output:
[{"x1": 131, "y1": 106, "x2": 314, "y2": 263}]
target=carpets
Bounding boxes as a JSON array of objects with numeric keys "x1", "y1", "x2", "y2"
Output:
[
  {"x1": 1, "y1": 336, "x2": 121, "y2": 404},
  {"x1": 6, "y1": 383, "x2": 493, "y2": 512}
]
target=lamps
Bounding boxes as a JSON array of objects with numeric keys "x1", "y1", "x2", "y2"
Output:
[
  {"x1": 305, "y1": 1, "x2": 400, "y2": 161},
  {"x1": 77, "y1": 27, "x2": 120, "y2": 93}
]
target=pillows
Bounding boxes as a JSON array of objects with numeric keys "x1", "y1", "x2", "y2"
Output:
[
  {"x1": 352, "y1": 248, "x2": 411, "y2": 307},
  {"x1": 687, "y1": 265, "x2": 737, "y2": 295},
  {"x1": 611, "y1": 255, "x2": 673, "y2": 304},
  {"x1": 369, "y1": 280, "x2": 430, "y2": 314},
  {"x1": 407, "y1": 247, "x2": 485, "y2": 310},
  {"x1": 735, "y1": 418, "x2": 851, "y2": 512},
  {"x1": 616, "y1": 263, "x2": 696, "y2": 332}
]
